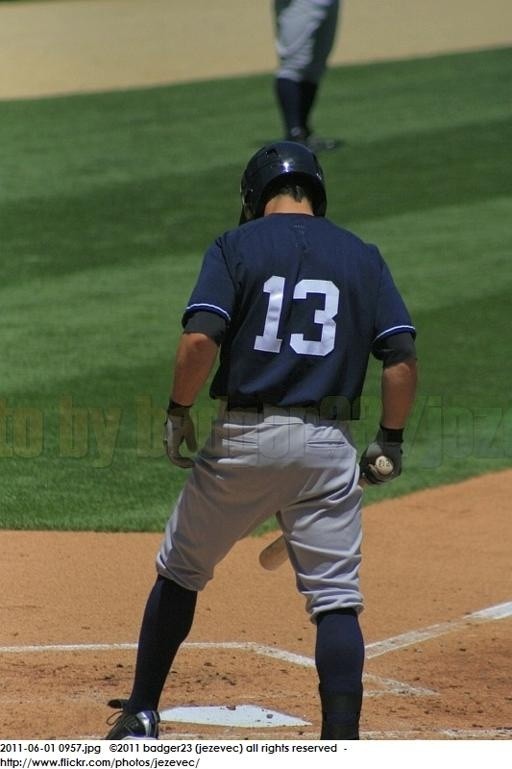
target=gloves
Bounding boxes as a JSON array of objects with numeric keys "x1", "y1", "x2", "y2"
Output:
[
  {"x1": 359, "y1": 423, "x2": 404, "y2": 486},
  {"x1": 166, "y1": 398, "x2": 197, "y2": 469}
]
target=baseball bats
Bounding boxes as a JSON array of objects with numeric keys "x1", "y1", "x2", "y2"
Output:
[{"x1": 259, "y1": 456, "x2": 394, "y2": 570}]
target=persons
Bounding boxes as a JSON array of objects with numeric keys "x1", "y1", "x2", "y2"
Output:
[
  {"x1": 272, "y1": 0, "x2": 340, "y2": 146},
  {"x1": 107, "y1": 141, "x2": 417, "y2": 740}
]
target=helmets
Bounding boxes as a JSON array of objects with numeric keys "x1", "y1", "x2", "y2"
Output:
[{"x1": 240, "y1": 141, "x2": 325, "y2": 215}]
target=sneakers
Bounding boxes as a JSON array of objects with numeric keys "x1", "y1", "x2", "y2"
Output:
[{"x1": 105, "y1": 708, "x2": 159, "y2": 740}]
